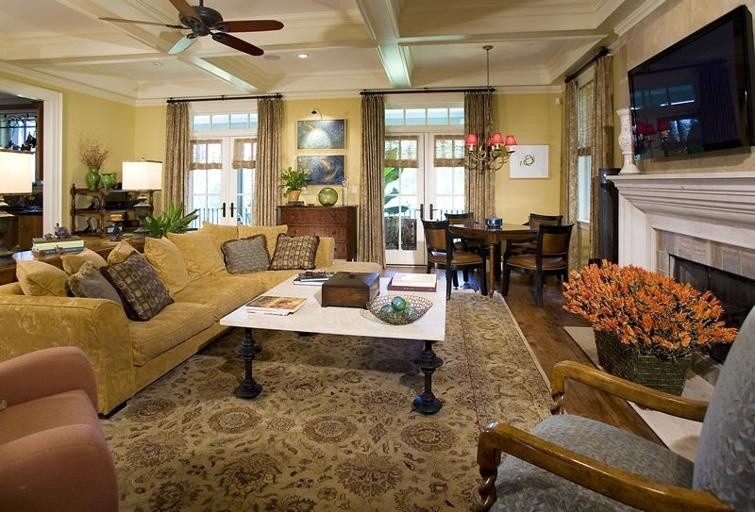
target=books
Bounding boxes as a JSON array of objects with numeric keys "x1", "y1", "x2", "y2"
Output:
[
  {"x1": 245, "y1": 296, "x2": 307, "y2": 316},
  {"x1": 31, "y1": 234, "x2": 84, "y2": 253},
  {"x1": 293, "y1": 270, "x2": 336, "y2": 285},
  {"x1": 387, "y1": 272, "x2": 437, "y2": 292}
]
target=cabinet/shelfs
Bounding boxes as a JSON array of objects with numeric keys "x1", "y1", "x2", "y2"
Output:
[
  {"x1": 70, "y1": 183, "x2": 138, "y2": 236},
  {"x1": 608, "y1": 109, "x2": 755, "y2": 387},
  {"x1": 0, "y1": 202, "x2": 43, "y2": 250},
  {"x1": 276, "y1": 204, "x2": 357, "y2": 260}
]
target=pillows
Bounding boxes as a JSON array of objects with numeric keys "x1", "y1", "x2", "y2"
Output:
[
  {"x1": 144, "y1": 236, "x2": 191, "y2": 294},
  {"x1": 269, "y1": 232, "x2": 321, "y2": 270},
  {"x1": 221, "y1": 234, "x2": 271, "y2": 274},
  {"x1": 202, "y1": 220, "x2": 238, "y2": 261},
  {"x1": 237, "y1": 224, "x2": 289, "y2": 260},
  {"x1": 68, "y1": 261, "x2": 124, "y2": 308},
  {"x1": 100, "y1": 250, "x2": 174, "y2": 321},
  {"x1": 166, "y1": 226, "x2": 226, "y2": 280},
  {"x1": 59, "y1": 247, "x2": 109, "y2": 276},
  {"x1": 107, "y1": 239, "x2": 142, "y2": 263},
  {"x1": 14, "y1": 257, "x2": 70, "y2": 297}
]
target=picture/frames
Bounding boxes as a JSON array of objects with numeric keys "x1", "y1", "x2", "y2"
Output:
[
  {"x1": 296, "y1": 154, "x2": 347, "y2": 187},
  {"x1": 295, "y1": 118, "x2": 347, "y2": 151},
  {"x1": 508, "y1": 144, "x2": 551, "y2": 179}
]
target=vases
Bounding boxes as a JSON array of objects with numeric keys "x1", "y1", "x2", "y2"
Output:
[
  {"x1": 100, "y1": 172, "x2": 117, "y2": 190},
  {"x1": 316, "y1": 183, "x2": 339, "y2": 206},
  {"x1": 590, "y1": 325, "x2": 693, "y2": 397}
]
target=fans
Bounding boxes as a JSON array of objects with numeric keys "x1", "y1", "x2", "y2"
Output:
[{"x1": 97, "y1": 0, "x2": 284, "y2": 57}]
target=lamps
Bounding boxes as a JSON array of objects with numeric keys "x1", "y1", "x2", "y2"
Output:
[
  {"x1": 311, "y1": 107, "x2": 323, "y2": 119},
  {"x1": 464, "y1": 45, "x2": 517, "y2": 171},
  {"x1": 121, "y1": 156, "x2": 163, "y2": 233},
  {"x1": 0, "y1": 148, "x2": 35, "y2": 197}
]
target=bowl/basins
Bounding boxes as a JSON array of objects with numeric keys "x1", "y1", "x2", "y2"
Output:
[{"x1": 366, "y1": 295, "x2": 433, "y2": 325}]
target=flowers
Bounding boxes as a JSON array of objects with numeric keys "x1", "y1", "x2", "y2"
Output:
[{"x1": 560, "y1": 258, "x2": 738, "y2": 357}]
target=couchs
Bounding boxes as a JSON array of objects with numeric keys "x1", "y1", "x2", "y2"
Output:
[
  {"x1": 0, "y1": 345, "x2": 120, "y2": 512},
  {"x1": 0, "y1": 235, "x2": 335, "y2": 416},
  {"x1": 476, "y1": 305, "x2": 755, "y2": 512}
]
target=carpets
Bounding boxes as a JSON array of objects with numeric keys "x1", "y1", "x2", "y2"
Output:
[
  {"x1": 562, "y1": 325, "x2": 714, "y2": 462},
  {"x1": 98, "y1": 288, "x2": 568, "y2": 512}
]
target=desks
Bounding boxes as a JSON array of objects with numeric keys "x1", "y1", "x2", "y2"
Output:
[
  {"x1": 219, "y1": 273, "x2": 447, "y2": 415},
  {"x1": 448, "y1": 222, "x2": 539, "y2": 298},
  {"x1": 0, "y1": 237, "x2": 146, "y2": 286}
]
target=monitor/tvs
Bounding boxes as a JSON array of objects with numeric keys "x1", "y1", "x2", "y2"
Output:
[{"x1": 626, "y1": 4, "x2": 755, "y2": 164}]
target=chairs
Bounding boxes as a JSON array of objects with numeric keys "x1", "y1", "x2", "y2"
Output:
[
  {"x1": 502, "y1": 222, "x2": 575, "y2": 307},
  {"x1": 503, "y1": 212, "x2": 563, "y2": 285},
  {"x1": 421, "y1": 219, "x2": 488, "y2": 300},
  {"x1": 444, "y1": 212, "x2": 481, "y2": 282}
]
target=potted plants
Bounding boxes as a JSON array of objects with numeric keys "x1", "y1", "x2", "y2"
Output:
[
  {"x1": 277, "y1": 166, "x2": 308, "y2": 201},
  {"x1": 79, "y1": 140, "x2": 109, "y2": 190}
]
